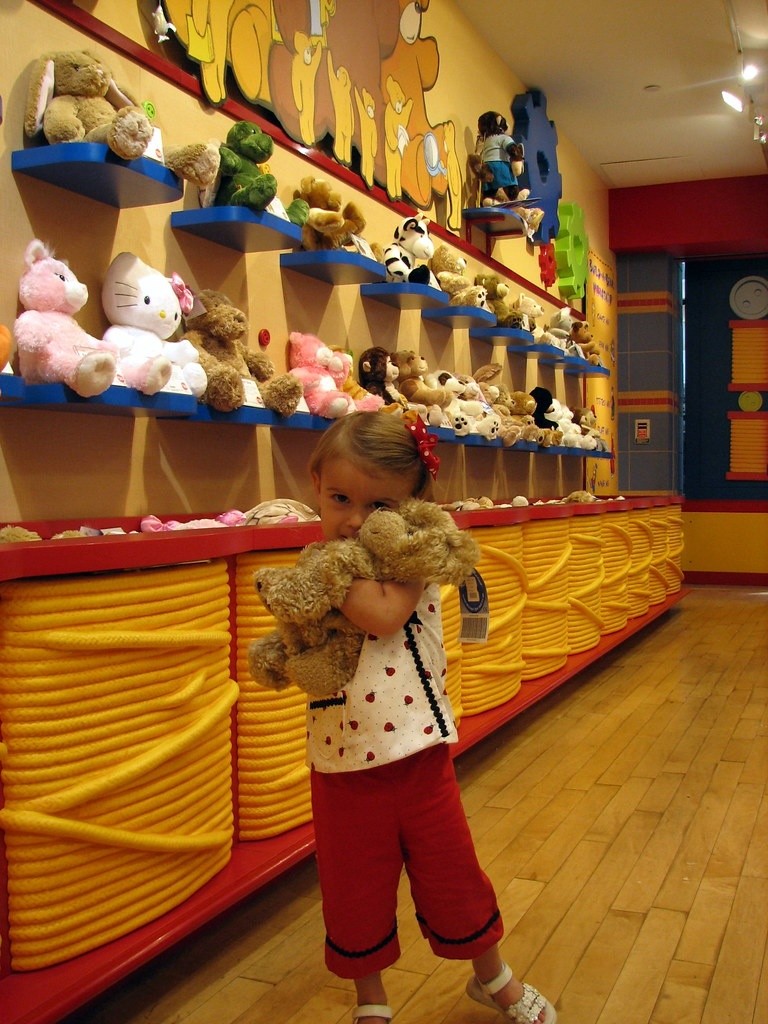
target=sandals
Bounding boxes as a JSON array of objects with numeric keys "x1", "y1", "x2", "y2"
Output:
[
  {"x1": 351, "y1": 1002, "x2": 393, "y2": 1024},
  {"x1": 465, "y1": 964, "x2": 556, "y2": 1024}
]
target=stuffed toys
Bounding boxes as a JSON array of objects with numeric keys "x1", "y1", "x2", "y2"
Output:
[
  {"x1": 248, "y1": 501, "x2": 478, "y2": 696},
  {"x1": 438, "y1": 491, "x2": 627, "y2": 511},
  {"x1": 1, "y1": 46, "x2": 611, "y2": 449},
  {"x1": 0, "y1": 498, "x2": 320, "y2": 544}
]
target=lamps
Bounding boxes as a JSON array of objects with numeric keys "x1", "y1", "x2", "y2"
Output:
[{"x1": 721, "y1": 87, "x2": 745, "y2": 111}]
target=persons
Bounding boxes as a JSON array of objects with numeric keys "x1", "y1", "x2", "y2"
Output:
[{"x1": 303, "y1": 411, "x2": 557, "y2": 1024}]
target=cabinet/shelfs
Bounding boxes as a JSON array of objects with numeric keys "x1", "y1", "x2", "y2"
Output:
[{"x1": 1, "y1": 144, "x2": 615, "y2": 458}]
